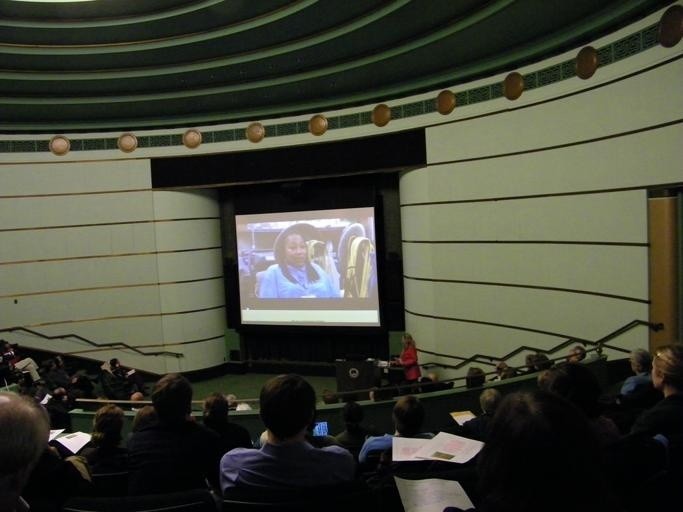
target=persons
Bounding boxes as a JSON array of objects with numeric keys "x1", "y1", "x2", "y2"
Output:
[
  {"x1": 259, "y1": 228, "x2": 341, "y2": 298},
  {"x1": 391, "y1": 333, "x2": 420, "y2": 382}
]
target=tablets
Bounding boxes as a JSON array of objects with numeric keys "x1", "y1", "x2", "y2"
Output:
[{"x1": 312, "y1": 421, "x2": 329, "y2": 439}]
